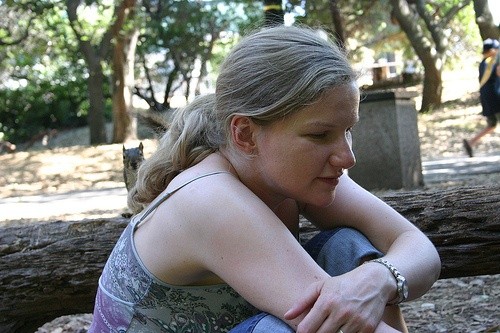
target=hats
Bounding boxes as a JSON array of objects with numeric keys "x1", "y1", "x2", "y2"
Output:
[{"x1": 484, "y1": 38, "x2": 499, "y2": 49}]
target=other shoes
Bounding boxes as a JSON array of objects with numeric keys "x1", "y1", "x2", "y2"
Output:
[{"x1": 462, "y1": 138, "x2": 473, "y2": 157}]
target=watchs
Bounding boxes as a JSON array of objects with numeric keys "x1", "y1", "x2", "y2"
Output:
[{"x1": 363, "y1": 258, "x2": 409, "y2": 306}]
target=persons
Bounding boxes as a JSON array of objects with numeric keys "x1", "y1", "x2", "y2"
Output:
[
  {"x1": 463, "y1": 37, "x2": 500, "y2": 158},
  {"x1": 88, "y1": 22, "x2": 441, "y2": 333}
]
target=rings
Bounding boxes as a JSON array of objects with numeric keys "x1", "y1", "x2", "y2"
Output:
[{"x1": 339, "y1": 329, "x2": 344, "y2": 333}]
